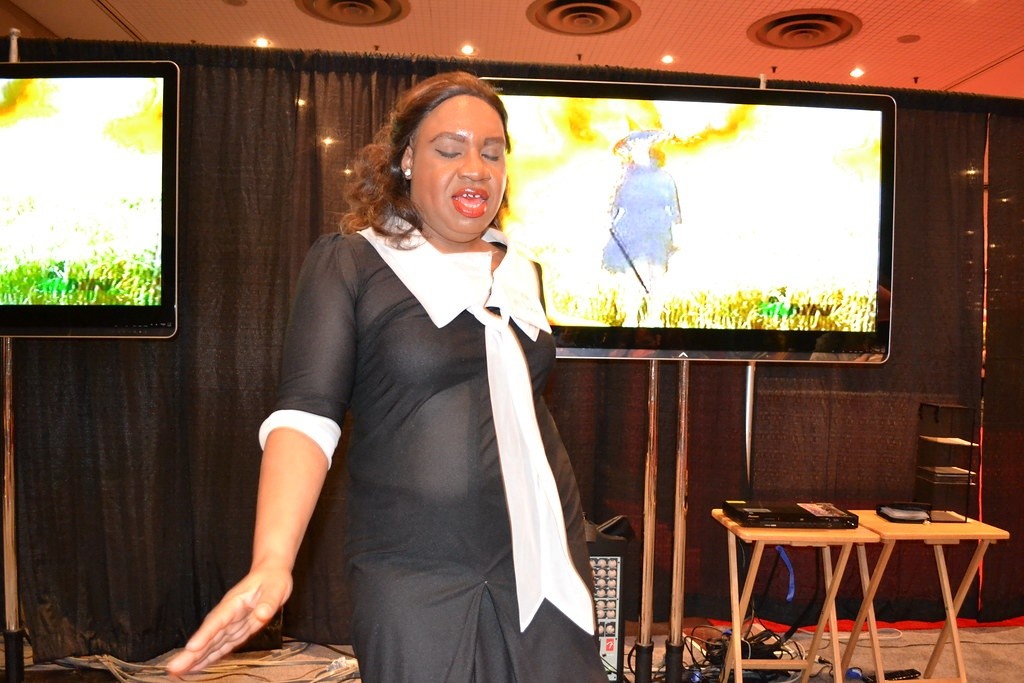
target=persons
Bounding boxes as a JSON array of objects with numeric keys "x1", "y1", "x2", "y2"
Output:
[{"x1": 167, "y1": 71, "x2": 608, "y2": 683}]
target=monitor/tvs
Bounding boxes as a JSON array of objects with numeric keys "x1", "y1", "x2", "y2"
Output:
[
  {"x1": 0, "y1": 60, "x2": 181, "y2": 336},
  {"x1": 481, "y1": 78, "x2": 897, "y2": 365}
]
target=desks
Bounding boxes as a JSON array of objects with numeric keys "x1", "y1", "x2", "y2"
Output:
[
  {"x1": 841, "y1": 509, "x2": 1011, "y2": 682},
  {"x1": 710, "y1": 507, "x2": 881, "y2": 682}
]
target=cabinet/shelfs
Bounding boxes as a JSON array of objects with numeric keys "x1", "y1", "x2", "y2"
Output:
[{"x1": 915, "y1": 400, "x2": 974, "y2": 523}]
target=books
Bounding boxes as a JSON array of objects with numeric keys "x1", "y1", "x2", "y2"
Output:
[{"x1": 919, "y1": 465, "x2": 976, "y2": 484}]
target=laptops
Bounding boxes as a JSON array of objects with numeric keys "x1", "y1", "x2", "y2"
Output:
[{"x1": 723, "y1": 500, "x2": 858, "y2": 529}]
